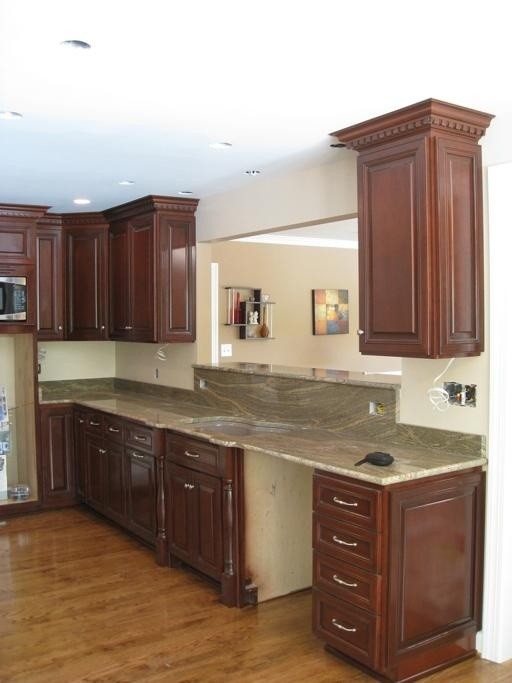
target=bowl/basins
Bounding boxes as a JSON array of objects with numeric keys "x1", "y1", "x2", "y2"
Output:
[{"x1": 261, "y1": 292, "x2": 271, "y2": 302}]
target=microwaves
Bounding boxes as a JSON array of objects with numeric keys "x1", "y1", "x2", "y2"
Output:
[{"x1": 0, "y1": 276, "x2": 27, "y2": 323}]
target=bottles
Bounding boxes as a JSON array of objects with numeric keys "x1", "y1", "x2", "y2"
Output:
[{"x1": 261, "y1": 323, "x2": 270, "y2": 338}]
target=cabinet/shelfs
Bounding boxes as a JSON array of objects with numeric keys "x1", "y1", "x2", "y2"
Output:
[
  {"x1": 1, "y1": 216, "x2": 40, "y2": 518},
  {"x1": 312, "y1": 468, "x2": 485, "y2": 683},
  {"x1": 38, "y1": 222, "x2": 111, "y2": 341},
  {"x1": 224, "y1": 286, "x2": 276, "y2": 340},
  {"x1": 40, "y1": 403, "x2": 313, "y2": 608},
  {"x1": 110, "y1": 210, "x2": 196, "y2": 344},
  {"x1": 356, "y1": 128, "x2": 484, "y2": 360}
]
